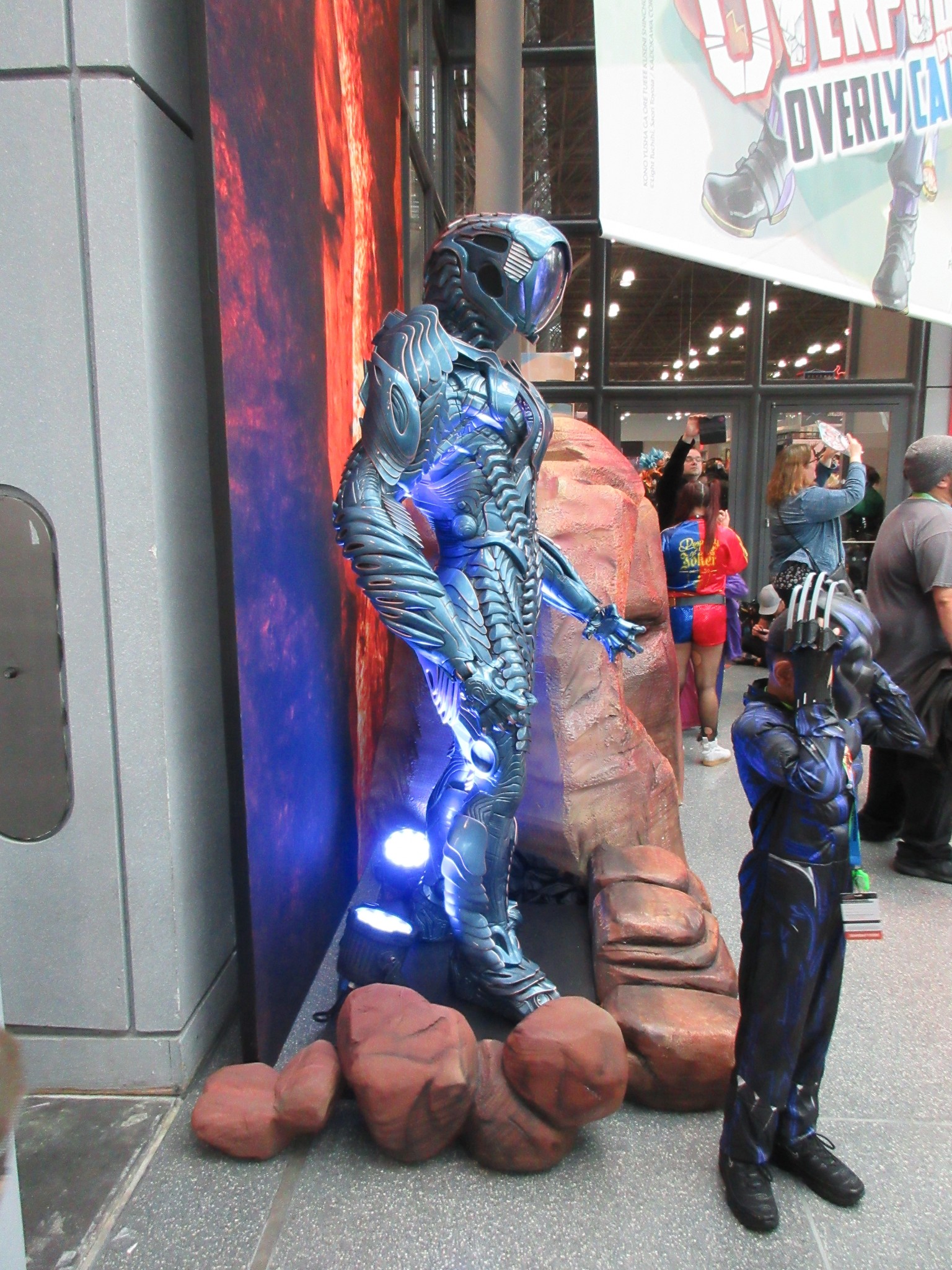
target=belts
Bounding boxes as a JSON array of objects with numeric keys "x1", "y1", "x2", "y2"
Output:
[{"x1": 668, "y1": 593, "x2": 726, "y2": 608}]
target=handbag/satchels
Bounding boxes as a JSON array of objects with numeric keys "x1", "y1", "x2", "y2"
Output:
[{"x1": 772, "y1": 562, "x2": 817, "y2": 600}]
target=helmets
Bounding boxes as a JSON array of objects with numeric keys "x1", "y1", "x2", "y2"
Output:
[
  {"x1": 786, "y1": 573, "x2": 883, "y2": 722},
  {"x1": 421, "y1": 212, "x2": 573, "y2": 352}
]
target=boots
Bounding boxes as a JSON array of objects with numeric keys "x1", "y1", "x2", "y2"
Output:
[{"x1": 701, "y1": 736, "x2": 731, "y2": 767}]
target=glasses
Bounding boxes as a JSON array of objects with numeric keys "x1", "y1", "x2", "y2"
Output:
[
  {"x1": 685, "y1": 456, "x2": 703, "y2": 464},
  {"x1": 808, "y1": 457, "x2": 818, "y2": 463}
]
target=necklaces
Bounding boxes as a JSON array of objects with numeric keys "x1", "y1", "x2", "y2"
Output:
[{"x1": 912, "y1": 492, "x2": 941, "y2": 503}]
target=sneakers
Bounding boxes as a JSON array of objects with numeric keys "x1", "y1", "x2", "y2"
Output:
[
  {"x1": 857, "y1": 810, "x2": 895, "y2": 842},
  {"x1": 769, "y1": 1132, "x2": 865, "y2": 1207},
  {"x1": 717, "y1": 1149, "x2": 781, "y2": 1230},
  {"x1": 894, "y1": 840, "x2": 952, "y2": 883}
]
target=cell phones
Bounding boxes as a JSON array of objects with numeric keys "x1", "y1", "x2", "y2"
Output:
[{"x1": 698, "y1": 414, "x2": 727, "y2": 445}]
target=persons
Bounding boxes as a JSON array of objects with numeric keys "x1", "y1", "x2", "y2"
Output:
[
  {"x1": 329, "y1": 210, "x2": 648, "y2": 1021},
  {"x1": 655, "y1": 414, "x2": 704, "y2": 533},
  {"x1": 860, "y1": 435, "x2": 952, "y2": 884},
  {"x1": 644, "y1": 442, "x2": 886, "y2": 540},
  {"x1": 661, "y1": 480, "x2": 749, "y2": 767},
  {"x1": 745, "y1": 583, "x2": 786, "y2": 668},
  {"x1": 766, "y1": 433, "x2": 867, "y2": 608},
  {"x1": 718, "y1": 572, "x2": 927, "y2": 1231}
]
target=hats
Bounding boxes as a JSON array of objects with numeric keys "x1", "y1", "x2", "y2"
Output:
[
  {"x1": 757, "y1": 584, "x2": 782, "y2": 615},
  {"x1": 905, "y1": 434, "x2": 952, "y2": 492}
]
